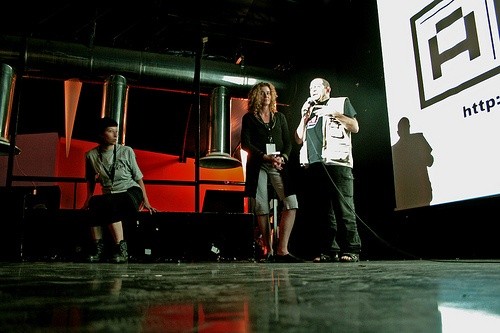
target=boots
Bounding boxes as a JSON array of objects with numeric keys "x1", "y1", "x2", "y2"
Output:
[
  {"x1": 110, "y1": 240, "x2": 129, "y2": 264},
  {"x1": 86, "y1": 238, "x2": 105, "y2": 263}
]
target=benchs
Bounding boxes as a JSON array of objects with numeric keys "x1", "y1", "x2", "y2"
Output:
[{"x1": 0, "y1": 212, "x2": 256, "y2": 262}]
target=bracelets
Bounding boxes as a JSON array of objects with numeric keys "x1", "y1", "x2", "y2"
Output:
[{"x1": 281, "y1": 157, "x2": 285, "y2": 162}]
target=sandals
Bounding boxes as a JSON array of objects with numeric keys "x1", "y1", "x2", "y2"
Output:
[
  {"x1": 313, "y1": 251, "x2": 340, "y2": 263},
  {"x1": 339, "y1": 252, "x2": 359, "y2": 262}
]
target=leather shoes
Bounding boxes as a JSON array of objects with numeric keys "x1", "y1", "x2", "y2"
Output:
[
  {"x1": 257, "y1": 251, "x2": 276, "y2": 263},
  {"x1": 275, "y1": 252, "x2": 304, "y2": 263}
]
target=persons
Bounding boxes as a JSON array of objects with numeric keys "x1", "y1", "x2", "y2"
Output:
[
  {"x1": 78, "y1": 116, "x2": 161, "y2": 264},
  {"x1": 294, "y1": 77, "x2": 361, "y2": 264},
  {"x1": 240, "y1": 82, "x2": 307, "y2": 263}
]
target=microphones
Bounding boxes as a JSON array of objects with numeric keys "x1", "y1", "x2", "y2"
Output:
[{"x1": 303, "y1": 97, "x2": 313, "y2": 114}]
